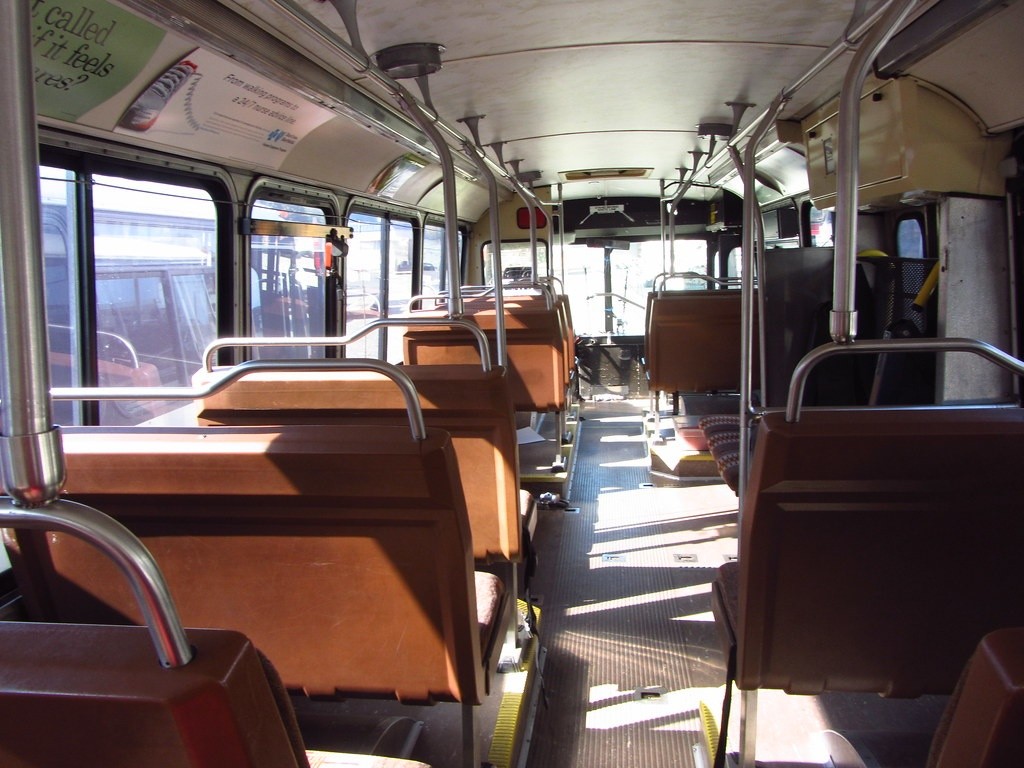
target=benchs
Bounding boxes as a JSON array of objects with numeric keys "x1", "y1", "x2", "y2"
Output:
[
  {"x1": 48, "y1": 322, "x2": 167, "y2": 413},
  {"x1": 642, "y1": 246, "x2": 1024, "y2": 768},
  {"x1": 0, "y1": 274, "x2": 577, "y2": 768}
]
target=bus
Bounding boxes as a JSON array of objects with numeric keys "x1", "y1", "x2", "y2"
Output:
[{"x1": 39, "y1": 164, "x2": 326, "y2": 428}]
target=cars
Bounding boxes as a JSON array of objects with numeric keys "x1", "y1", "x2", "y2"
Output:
[{"x1": 345, "y1": 258, "x2": 445, "y2": 321}]
